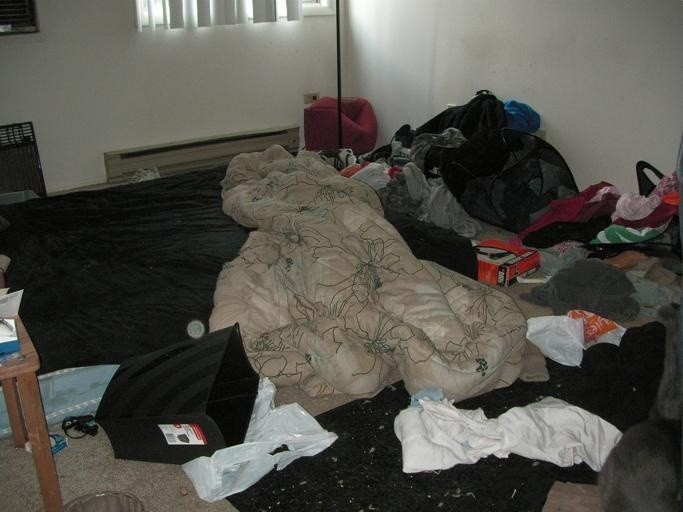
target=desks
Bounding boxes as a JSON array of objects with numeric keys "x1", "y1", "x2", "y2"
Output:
[{"x1": 0, "y1": 268, "x2": 60, "y2": 512}]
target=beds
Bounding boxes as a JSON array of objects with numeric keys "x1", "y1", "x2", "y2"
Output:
[{"x1": 0, "y1": 163, "x2": 482, "y2": 437}]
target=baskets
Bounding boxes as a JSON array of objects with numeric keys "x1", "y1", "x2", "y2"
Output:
[{"x1": 0, "y1": 122, "x2": 47, "y2": 206}]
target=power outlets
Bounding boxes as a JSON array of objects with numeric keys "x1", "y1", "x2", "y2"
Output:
[{"x1": 305, "y1": 91, "x2": 319, "y2": 103}]
